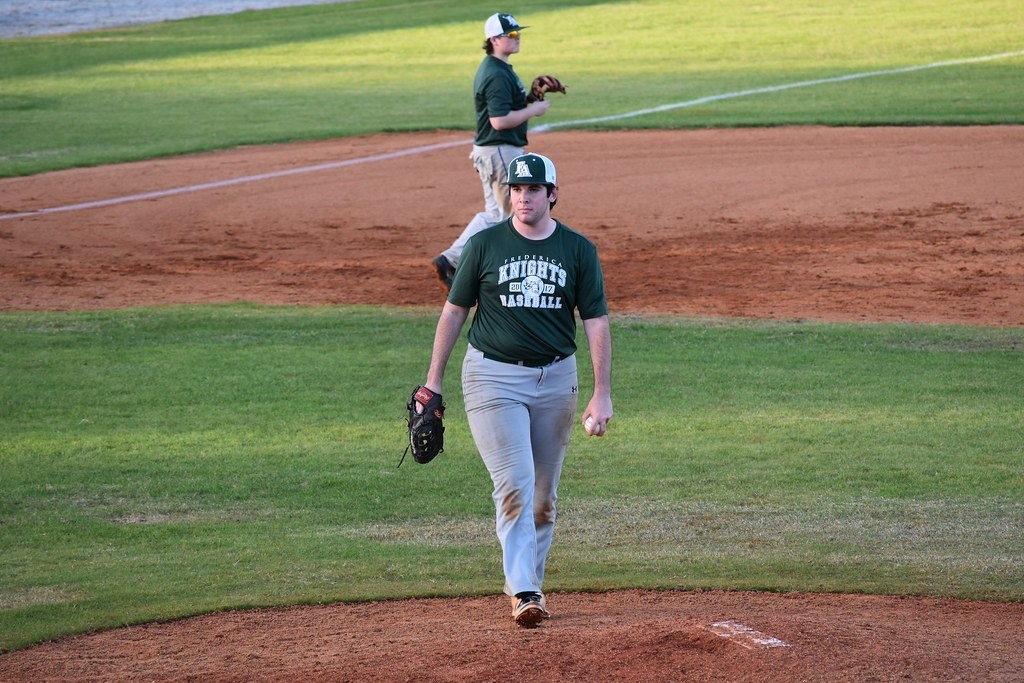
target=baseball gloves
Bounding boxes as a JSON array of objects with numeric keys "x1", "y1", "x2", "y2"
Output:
[
  {"x1": 525, "y1": 75, "x2": 569, "y2": 102},
  {"x1": 404, "y1": 384, "x2": 447, "y2": 465}
]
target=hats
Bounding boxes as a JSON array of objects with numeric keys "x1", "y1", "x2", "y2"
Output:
[
  {"x1": 485, "y1": 12, "x2": 529, "y2": 41},
  {"x1": 499, "y1": 152, "x2": 557, "y2": 188}
]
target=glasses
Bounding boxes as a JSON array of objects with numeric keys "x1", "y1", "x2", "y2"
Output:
[{"x1": 500, "y1": 31, "x2": 521, "y2": 38}]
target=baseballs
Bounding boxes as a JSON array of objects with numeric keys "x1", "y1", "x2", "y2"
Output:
[{"x1": 583, "y1": 416, "x2": 601, "y2": 435}]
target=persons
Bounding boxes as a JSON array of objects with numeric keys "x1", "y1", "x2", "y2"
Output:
[
  {"x1": 430, "y1": 10, "x2": 549, "y2": 296},
  {"x1": 411, "y1": 152, "x2": 619, "y2": 630}
]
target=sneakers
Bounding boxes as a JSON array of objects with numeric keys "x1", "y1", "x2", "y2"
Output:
[
  {"x1": 430, "y1": 255, "x2": 456, "y2": 298},
  {"x1": 509, "y1": 592, "x2": 551, "y2": 628}
]
